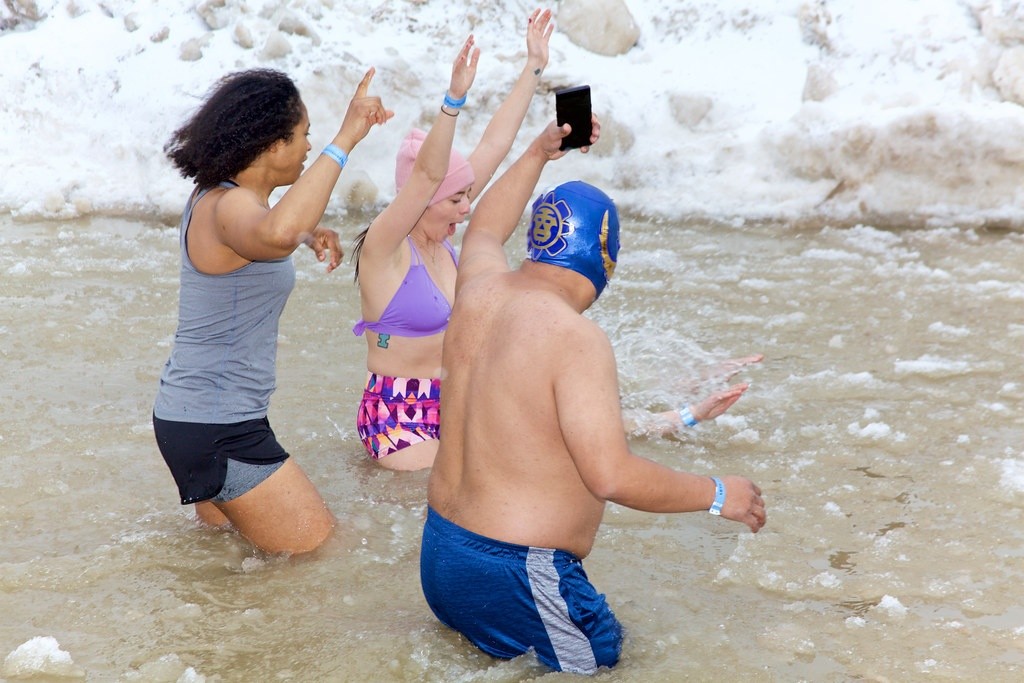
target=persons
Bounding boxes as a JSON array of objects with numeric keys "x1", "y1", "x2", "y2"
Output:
[
  {"x1": 349, "y1": 7, "x2": 555, "y2": 470},
  {"x1": 622, "y1": 353, "x2": 764, "y2": 434},
  {"x1": 419, "y1": 110, "x2": 765, "y2": 673},
  {"x1": 161, "y1": 65, "x2": 394, "y2": 556}
]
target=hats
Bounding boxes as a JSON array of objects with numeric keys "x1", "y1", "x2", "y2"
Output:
[
  {"x1": 396, "y1": 129, "x2": 475, "y2": 211},
  {"x1": 529, "y1": 182, "x2": 620, "y2": 308}
]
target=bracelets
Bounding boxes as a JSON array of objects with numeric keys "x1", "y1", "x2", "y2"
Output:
[
  {"x1": 709, "y1": 475, "x2": 725, "y2": 516},
  {"x1": 444, "y1": 89, "x2": 466, "y2": 109},
  {"x1": 441, "y1": 105, "x2": 460, "y2": 117},
  {"x1": 680, "y1": 408, "x2": 697, "y2": 427},
  {"x1": 322, "y1": 144, "x2": 349, "y2": 169}
]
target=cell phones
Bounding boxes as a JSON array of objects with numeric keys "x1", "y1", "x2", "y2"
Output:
[{"x1": 556, "y1": 84, "x2": 592, "y2": 152}]
